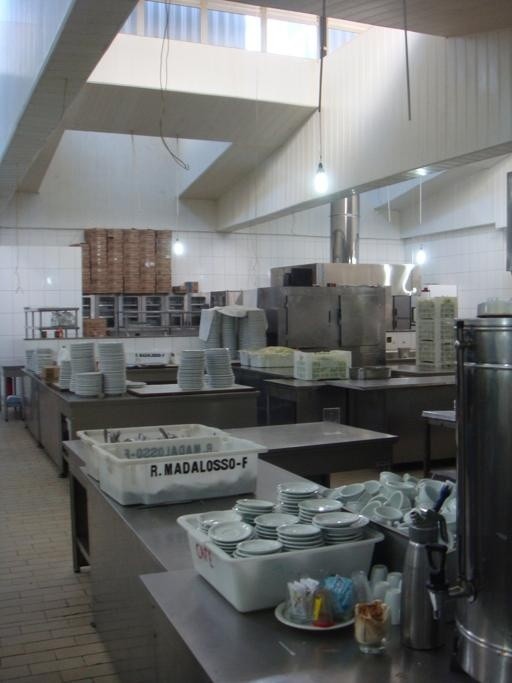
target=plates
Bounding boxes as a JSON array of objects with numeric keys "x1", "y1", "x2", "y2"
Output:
[
  {"x1": 197, "y1": 483, "x2": 370, "y2": 560},
  {"x1": 177, "y1": 347, "x2": 235, "y2": 392},
  {"x1": 273, "y1": 603, "x2": 355, "y2": 632},
  {"x1": 59, "y1": 342, "x2": 146, "y2": 397},
  {"x1": 25, "y1": 348, "x2": 54, "y2": 376},
  {"x1": 200, "y1": 308, "x2": 267, "y2": 349}
]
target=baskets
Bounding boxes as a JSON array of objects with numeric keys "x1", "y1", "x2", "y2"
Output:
[
  {"x1": 415, "y1": 294, "x2": 459, "y2": 369},
  {"x1": 291, "y1": 350, "x2": 353, "y2": 381},
  {"x1": 237, "y1": 348, "x2": 293, "y2": 368}
]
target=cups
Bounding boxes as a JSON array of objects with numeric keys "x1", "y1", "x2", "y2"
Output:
[
  {"x1": 351, "y1": 564, "x2": 402, "y2": 625},
  {"x1": 355, "y1": 612, "x2": 392, "y2": 656}
]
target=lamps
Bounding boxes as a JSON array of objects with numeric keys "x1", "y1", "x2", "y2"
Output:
[
  {"x1": 313, "y1": 0, "x2": 329, "y2": 195},
  {"x1": 415, "y1": 174, "x2": 426, "y2": 266}
]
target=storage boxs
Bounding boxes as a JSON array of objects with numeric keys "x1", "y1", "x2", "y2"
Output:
[{"x1": 80, "y1": 229, "x2": 199, "y2": 294}]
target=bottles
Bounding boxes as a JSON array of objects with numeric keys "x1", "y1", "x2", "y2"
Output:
[{"x1": 402, "y1": 506, "x2": 450, "y2": 651}]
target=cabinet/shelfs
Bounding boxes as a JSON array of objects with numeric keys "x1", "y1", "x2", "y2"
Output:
[
  {"x1": 83, "y1": 290, "x2": 245, "y2": 339},
  {"x1": 23, "y1": 307, "x2": 80, "y2": 341}
]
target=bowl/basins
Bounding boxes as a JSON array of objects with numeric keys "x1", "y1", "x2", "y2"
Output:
[{"x1": 336, "y1": 470, "x2": 457, "y2": 532}]
